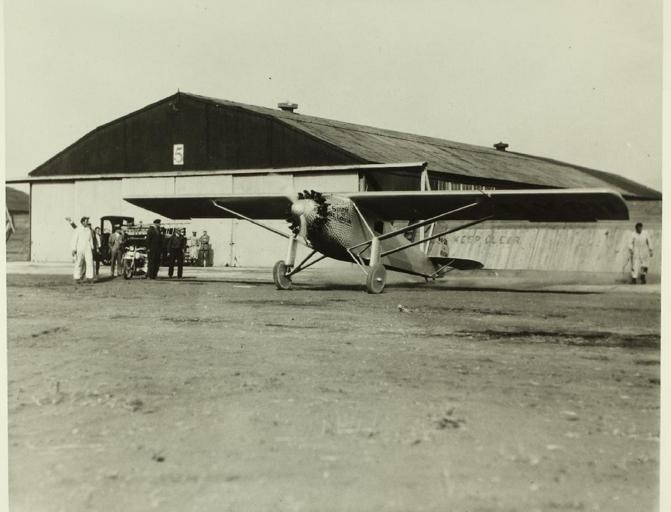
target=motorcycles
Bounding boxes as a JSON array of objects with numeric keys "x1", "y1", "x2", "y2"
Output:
[{"x1": 116, "y1": 241, "x2": 148, "y2": 280}]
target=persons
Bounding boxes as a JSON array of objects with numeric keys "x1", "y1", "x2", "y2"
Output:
[
  {"x1": 626, "y1": 223, "x2": 653, "y2": 284},
  {"x1": 66, "y1": 217, "x2": 210, "y2": 284}
]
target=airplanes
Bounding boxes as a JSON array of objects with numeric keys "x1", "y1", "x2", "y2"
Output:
[{"x1": 123, "y1": 189, "x2": 632, "y2": 295}]
